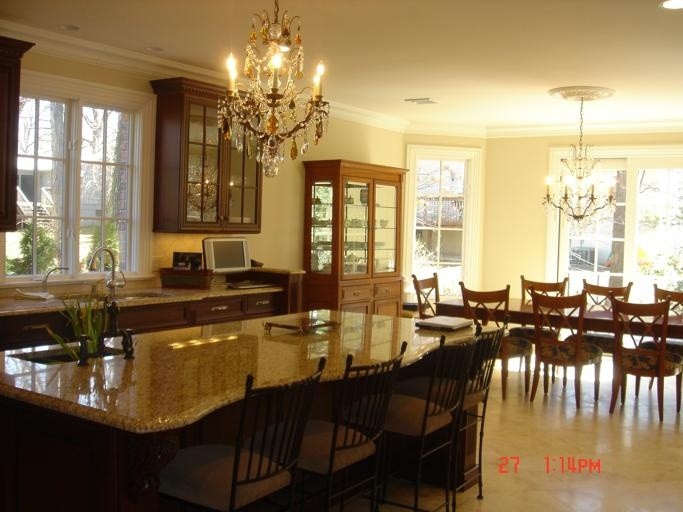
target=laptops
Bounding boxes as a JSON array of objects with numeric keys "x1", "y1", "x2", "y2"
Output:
[{"x1": 415, "y1": 315, "x2": 474, "y2": 333}]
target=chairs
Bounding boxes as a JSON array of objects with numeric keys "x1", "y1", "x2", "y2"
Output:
[
  {"x1": 410, "y1": 272, "x2": 439, "y2": 320},
  {"x1": 458, "y1": 279, "x2": 533, "y2": 399},
  {"x1": 342, "y1": 333, "x2": 480, "y2": 511},
  {"x1": 153, "y1": 352, "x2": 326, "y2": 509},
  {"x1": 526, "y1": 287, "x2": 604, "y2": 411},
  {"x1": 244, "y1": 336, "x2": 407, "y2": 512},
  {"x1": 510, "y1": 273, "x2": 567, "y2": 383},
  {"x1": 402, "y1": 311, "x2": 510, "y2": 512},
  {"x1": 607, "y1": 290, "x2": 682, "y2": 422},
  {"x1": 639, "y1": 284, "x2": 682, "y2": 389},
  {"x1": 564, "y1": 277, "x2": 634, "y2": 376}
]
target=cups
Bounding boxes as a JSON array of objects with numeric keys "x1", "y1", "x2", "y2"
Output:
[{"x1": 345, "y1": 218, "x2": 387, "y2": 228}]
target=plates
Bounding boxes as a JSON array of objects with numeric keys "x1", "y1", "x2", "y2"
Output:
[
  {"x1": 187, "y1": 214, "x2": 249, "y2": 223},
  {"x1": 357, "y1": 264, "x2": 365, "y2": 272}
]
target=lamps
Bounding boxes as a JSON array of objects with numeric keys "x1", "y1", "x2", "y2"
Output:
[{"x1": 214, "y1": 1, "x2": 328, "y2": 179}]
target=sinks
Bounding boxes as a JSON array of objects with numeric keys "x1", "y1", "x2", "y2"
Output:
[{"x1": 51, "y1": 289, "x2": 175, "y2": 302}]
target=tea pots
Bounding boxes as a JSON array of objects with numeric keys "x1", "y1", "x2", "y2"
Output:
[{"x1": 345, "y1": 253, "x2": 359, "y2": 272}]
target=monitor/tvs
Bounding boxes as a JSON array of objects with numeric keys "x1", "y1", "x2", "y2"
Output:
[{"x1": 202, "y1": 237, "x2": 252, "y2": 274}]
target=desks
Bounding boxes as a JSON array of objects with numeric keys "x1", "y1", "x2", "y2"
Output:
[
  {"x1": 540, "y1": 85, "x2": 619, "y2": 220},
  {"x1": 428, "y1": 293, "x2": 682, "y2": 349}
]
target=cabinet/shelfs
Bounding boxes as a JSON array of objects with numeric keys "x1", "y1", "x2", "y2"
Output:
[
  {"x1": 2, "y1": 311, "x2": 101, "y2": 355},
  {"x1": 299, "y1": 158, "x2": 409, "y2": 317},
  {"x1": 0, "y1": 36, "x2": 36, "y2": 233},
  {"x1": 191, "y1": 292, "x2": 283, "y2": 324},
  {"x1": 146, "y1": 76, "x2": 262, "y2": 235},
  {"x1": 101, "y1": 301, "x2": 191, "y2": 332}
]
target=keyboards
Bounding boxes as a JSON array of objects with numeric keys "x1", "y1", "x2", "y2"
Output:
[{"x1": 227, "y1": 280, "x2": 281, "y2": 289}]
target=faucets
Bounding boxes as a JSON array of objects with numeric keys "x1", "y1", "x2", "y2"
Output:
[
  {"x1": 88, "y1": 246, "x2": 127, "y2": 298},
  {"x1": 41, "y1": 267, "x2": 70, "y2": 291}
]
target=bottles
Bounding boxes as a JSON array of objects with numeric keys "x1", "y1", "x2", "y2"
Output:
[
  {"x1": 359, "y1": 187, "x2": 368, "y2": 203},
  {"x1": 346, "y1": 195, "x2": 353, "y2": 204},
  {"x1": 314, "y1": 196, "x2": 320, "y2": 203}
]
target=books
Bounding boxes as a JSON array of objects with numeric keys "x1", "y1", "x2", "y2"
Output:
[{"x1": 415, "y1": 314, "x2": 474, "y2": 333}]
